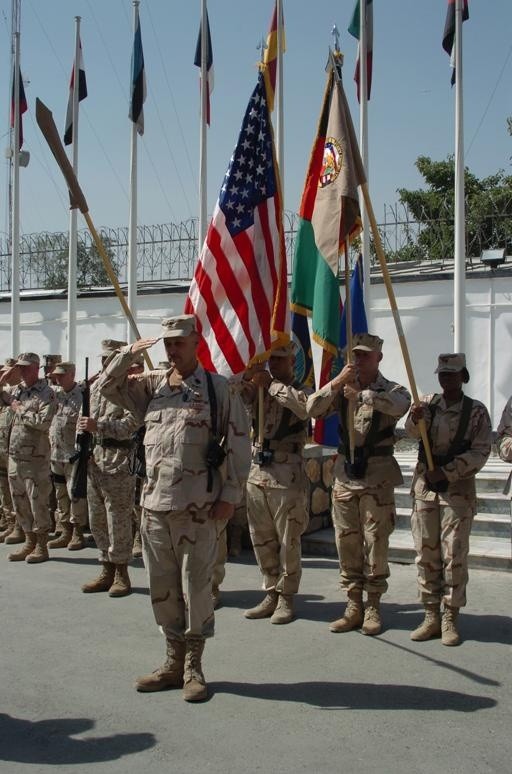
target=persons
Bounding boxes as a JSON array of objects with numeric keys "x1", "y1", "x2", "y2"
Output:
[
  {"x1": 305, "y1": 333, "x2": 411, "y2": 635},
  {"x1": 98, "y1": 313, "x2": 252, "y2": 703},
  {"x1": 491, "y1": 390, "x2": 511, "y2": 504},
  {"x1": 1, "y1": 331, "x2": 256, "y2": 598},
  {"x1": 226, "y1": 341, "x2": 312, "y2": 623},
  {"x1": 405, "y1": 351, "x2": 492, "y2": 645}
]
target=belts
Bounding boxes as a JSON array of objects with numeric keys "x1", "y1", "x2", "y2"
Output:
[
  {"x1": 337, "y1": 444, "x2": 394, "y2": 456},
  {"x1": 92, "y1": 437, "x2": 137, "y2": 449},
  {"x1": 417, "y1": 453, "x2": 454, "y2": 467},
  {"x1": 255, "y1": 437, "x2": 304, "y2": 457}
]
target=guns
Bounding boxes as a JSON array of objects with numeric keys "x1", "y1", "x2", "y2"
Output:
[{"x1": 69, "y1": 357, "x2": 101, "y2": 498}]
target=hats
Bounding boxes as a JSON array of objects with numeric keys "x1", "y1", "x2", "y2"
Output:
[
  {"x1": 15, "y1": 352, "x2": 40, "y2": 366},
  {"x1": 434, "y1": 352, "x2": 466, "y2": 374},
  {"x1": 351, "y1": 334, "x2": 383, "y2": 352},
  {"x1": 0, "y1": 358, "x2": 18, "y2": 372},
  {"x1": 97, "y1": 339, "x2": 128, "y2": 357},
  {"x1": 39, "y1": 355, "x2": 62, "y2": 368},
  {"x1": 154, "y1": 361, "x2": 170, "y2": 370},
  {"x1": 51, "y1": 362, "x2": 76, "y2": 375},
  {"x1": 127, "y1": 356, "x2": 145, "y2": 368},
  {"x1": 270, "y1": 341, "x2": 298, "y2": 357},
  {"x1": 161, "y1": 314, "x2": 198, "y2": 339}
]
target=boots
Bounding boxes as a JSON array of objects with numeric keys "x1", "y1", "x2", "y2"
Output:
[
  {"x1": 7, "y1": 532, "x2": 49, "y2": 564},
  {"x1": 211, "y1": 584, "x2": 220, "y2": 610},
  {"x1": 81, "y1": 561, "x2": 115, "y2": 593},
  {"x1": 270, "y1": 592, "x2": 298, "y2": 624},
  {"x1": 410, "y1": 602, "x2": 442, "y2": 641},
  {"x1": 135, "y1": 637, "x2": 186, "y2": 692},
  {"x1": 440, "y1": 603, "x2": 460, "y2": 646},
  {"x1": 362, "y1": 591, "x2": 383, "y2": 635},
  {"x1": 183, "y1": 638, "x2": 208, "y2": 701},
  {"x1": 329, "y1": 590, "x2": 365, "y2": 632},
  {"x1": 243, "y1": 589, "x2": 280, "y2": 619},
  {"x1": 0, "y1": 510, "x2": 144, "y2": 557},
  {"x1": 108, "y1": 563, "x2": 131, "y2": 597}
]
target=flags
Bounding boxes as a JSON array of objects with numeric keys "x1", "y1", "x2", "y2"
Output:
[
  {"x1": 128, "y1": 7, "x2": 148, "y2": 135},
  {"x1": 348, "y1": 0, "x2": 374, "y2": 104},
  {"x1": 64, "y1": 32, "x2": 87, "y2": 145},
  {"x1": 290, "y1": 69, "x2": 368, "y2": 446},
  {"x1": 264, "y1": 0, "x2": 287, "y2": 112},
  {"x1": 441, "y1": 0, "x2": 469, "y2": 89},
  {"x1": 9, "y1": 47, "x2": 28, "y2": 150},
  {"x1": 180, "y1": 62, "x2": 282, "y2": 378},
  {"x1": 192, "y1": 6, "x2": 216, "y2": 128}
]
